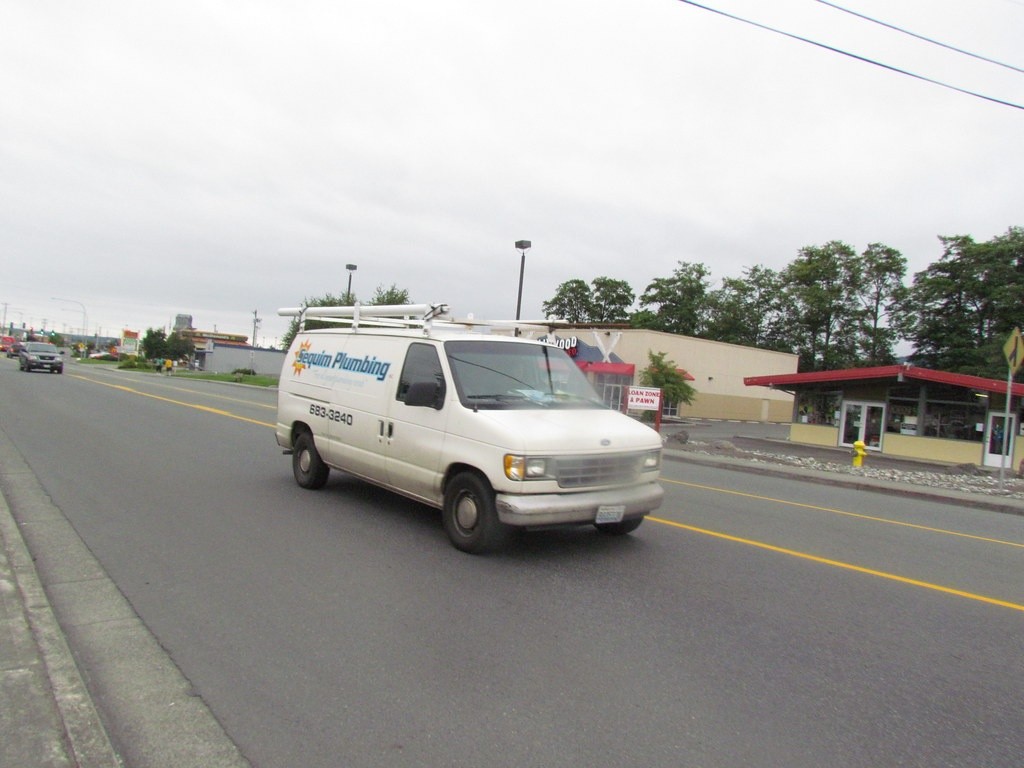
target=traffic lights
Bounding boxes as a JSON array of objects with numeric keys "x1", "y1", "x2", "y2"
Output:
[
  {"x1": 40, "y1": 329, "x2": 43, "y2": 335},
  {"x1": 52, "y1": 330, "x2": 54, "y2": 336}
]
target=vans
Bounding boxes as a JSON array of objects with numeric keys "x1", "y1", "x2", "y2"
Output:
[{"x1": 276, "y1": 304, "x2": 664, "y2": 554}]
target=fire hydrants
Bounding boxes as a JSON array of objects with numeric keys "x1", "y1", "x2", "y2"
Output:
[{"x1": 851, "y1": 441, "x2": 866, "y2": 466}]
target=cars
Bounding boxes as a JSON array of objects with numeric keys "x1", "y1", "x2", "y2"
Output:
[
  {"x1": 19, "y1": 342, "x2": 63, "y2": 373},
  {"x1": 0, "y1": 335, "x2": 15, "y2": 352},
  {"x1": 6, "y1": 344, "x2": 24, "y2": 357}
]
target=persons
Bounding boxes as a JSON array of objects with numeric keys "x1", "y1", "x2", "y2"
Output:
[{"x1": 146, "y1": 357, "x2": 184, "y2": 377}]
